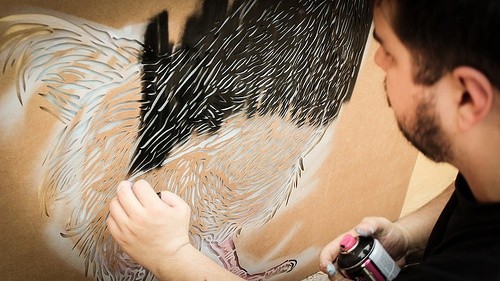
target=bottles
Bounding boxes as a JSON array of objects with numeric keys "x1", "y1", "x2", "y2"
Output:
[{"x1": 338, "y1": 234, "x2": 400, "y2": 281}]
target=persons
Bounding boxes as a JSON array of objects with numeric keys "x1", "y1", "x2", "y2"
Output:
[{"x1": 105, "y1": 0, "x2": 500, "y2": 280}]
